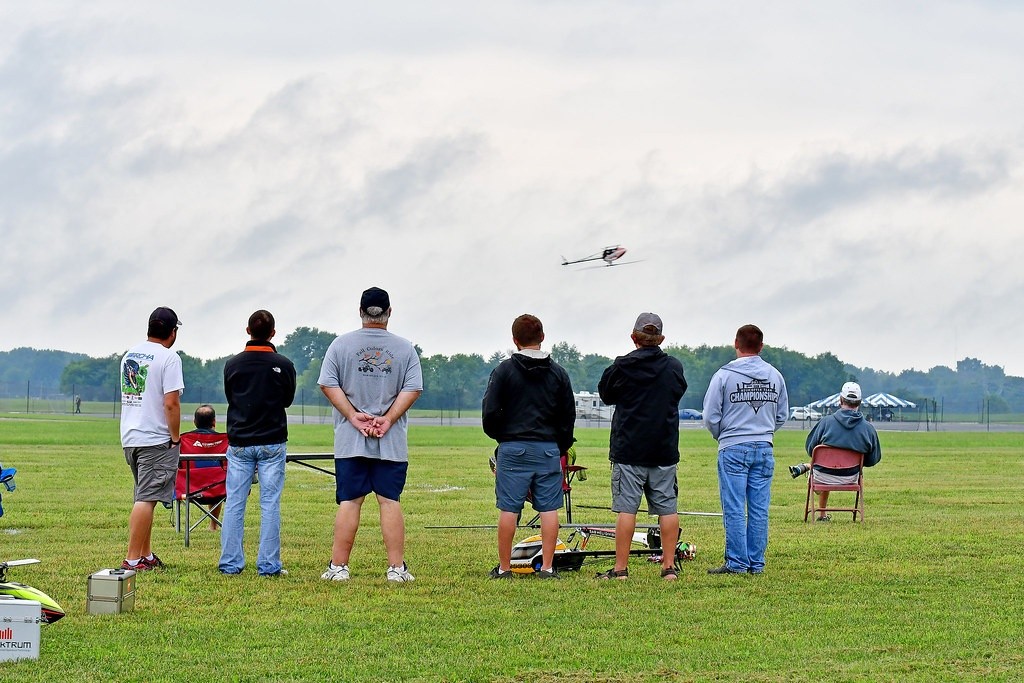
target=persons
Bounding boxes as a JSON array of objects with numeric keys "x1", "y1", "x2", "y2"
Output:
[
  {"x1": 119, "y1": 307, "x2": 185, "y2": 572},
  {"x1": 789, "y1": 381, "x2": 882, "y2": 522},
  {"x1": 194, "y1": 405, "x2": 222, "y2": 529},
  {"x1": 593, "y1": 312, "x2": 688, "y2": 580},
  {"x1": 218, "y1": 310, "x2": 288, "y2": 579},
  {"x1": 703, "y1": 325, "x2": 789, "y2": 574},
  {"x1": 316, "y1": 287, "x2": 424, "y2": 582},
  {"x1": 76, "y1": 396, "x2": 81, "y2": 413},
  {"x1": 481, "y1": 314, "x2": 576, "y2": 580}
]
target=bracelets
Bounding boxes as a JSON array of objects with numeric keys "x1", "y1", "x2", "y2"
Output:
[{"x1": 172, "y1": 438, "x2": 181, "y2": 445}]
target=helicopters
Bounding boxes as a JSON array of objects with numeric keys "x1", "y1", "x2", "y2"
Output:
[
  {"x1": 1, "y1": 558, "x2": 71, "y2": 627},
  {"x1": 421, "y1": 441, "x2": 728, "y2": 578},
  {"x1": 561, "y1": 243, "x2": 647, "y2": 272}
]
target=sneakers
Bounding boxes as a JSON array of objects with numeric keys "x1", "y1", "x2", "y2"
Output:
[
  {"x1": 387, "y1": 562, "x2": 415, "y2": 581},
  {"x1": 539, "y1": 567, "x2": 560, "y2": 578},
  {"x1": 142, "y1": 553, "x2": 163, "y2": 566},
  {"x1": 321, "y1": 560, "x2": 350, "y2": 580},
  {"x1": 490, "y1": 563, "x2": 513, "y2": 578},
  {"x1": 121, "y1": 558, "x2": 153, "y2": 571}
]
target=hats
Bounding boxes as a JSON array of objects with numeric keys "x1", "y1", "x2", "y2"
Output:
[
  {"x1": 635, "y1": 312, "x2": 662, "y2": 335},
  {"x1": 150, "y1": 306, "x2": 182, "y2": 331},
  {"x1": 840, "y1": 382, "x2": 862, "y2": 401},
  {"x1": 361, "y1": 287, "x2": 390, "y2": 318}
]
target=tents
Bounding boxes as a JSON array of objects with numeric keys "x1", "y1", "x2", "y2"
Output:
[
  {"x1": 807, "y1": 392, "x2": 878, "y2": 409},
  {"x1": 865, "y1": 392, "x2": 918, "y2": 409}
]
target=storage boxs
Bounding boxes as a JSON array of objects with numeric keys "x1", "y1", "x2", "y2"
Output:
[
  {"x1": 86, "y1": 568, "x2": 138, "y2": 616},
  {"x1": 0, "y1": 599, "x2": 43, "y2": 666}
]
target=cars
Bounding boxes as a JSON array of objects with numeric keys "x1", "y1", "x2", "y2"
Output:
[
  {"x1": 789, "y1": 406, "x2": 823, "y2": 421},
  {"x1": 679, "y1": 409, "x2": 703, "y2": 421}
]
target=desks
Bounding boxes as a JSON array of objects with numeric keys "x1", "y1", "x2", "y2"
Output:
[{"x1": 175, "y1": 450, "x2": 338, "y2": 549}]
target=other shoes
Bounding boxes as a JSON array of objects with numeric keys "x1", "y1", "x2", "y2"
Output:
[
  {"x1": 707, "y1": 565, "x2": 735, "y2": 574},
  {"x1": 788, "y1": 463, "x2": 808, "y2": 478},
  {"x1": 279, "y1": 569, "x2": 288, "y2": 577},
  {"x1": 816, "y1": 516, "x2": 830, "y2": 522}
]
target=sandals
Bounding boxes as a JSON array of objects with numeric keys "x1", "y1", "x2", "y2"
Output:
[
  {"x1": 594, "y1": 567, "x2": 628, "y2": 580},
  {"x1": 660, "y1": 566, "x2": 678, "y2": 580}
]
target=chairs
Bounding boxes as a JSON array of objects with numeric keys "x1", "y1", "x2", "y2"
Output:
[
  {"x1": 486, "y1": 439, "x2": 584, "y2": 526},
  {"x1": 0, "y1": 467, "x2": 17, "y2": 517},
  {"x1": 167, "y1": 432, "x2": 229, "y2": 529},
  {"x1": 804, "y1": 445, "x2": 867, "y2": 526}
]
